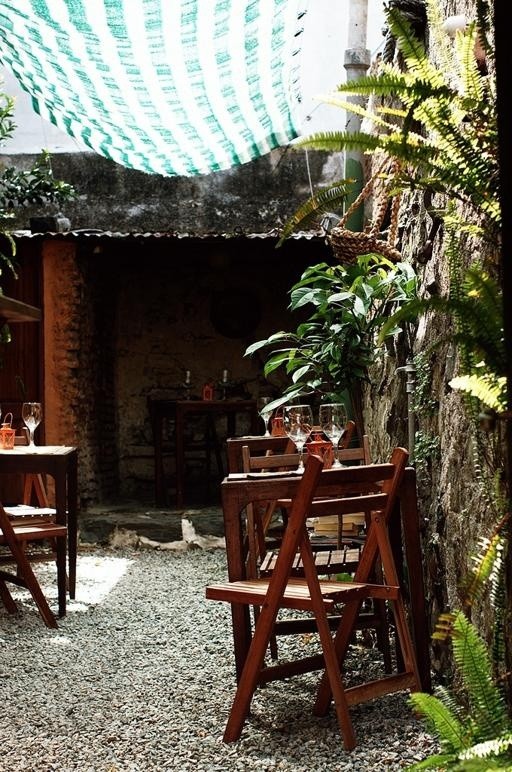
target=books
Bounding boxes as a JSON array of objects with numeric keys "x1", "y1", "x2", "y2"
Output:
[{"x1": 314, "y1": 511, "x2": 367, "y2": 537}]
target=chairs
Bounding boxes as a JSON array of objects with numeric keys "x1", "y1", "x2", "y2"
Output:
[
  {"x1": 0, "y1": 503, "x2": 67, "y2": 629},
  {"x1": 146, "y1": 395, "x2": 224, "y2": 506},
  {"x1": 240, "y1": 442, "x2": 393, "y2": 674},
  {"x1": 204, "y1": 446, "x2": 420, "y2": 750}
]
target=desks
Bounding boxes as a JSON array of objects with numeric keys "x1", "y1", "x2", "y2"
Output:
[
  {"x1": 1, "y1": 444, "x2": 79, "y2": 618},
  {"x1": 174, "y1": 399, "x2": 262, "y2": 507},
  {"x1": 221, "y1": 471, "x2": 434, "y2": 697}
]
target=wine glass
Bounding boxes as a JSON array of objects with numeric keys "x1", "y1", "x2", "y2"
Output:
[
  {"x1": 22, "y1": 402, "x2": 43, "y2": 451},
  {"x1": 257, "y1": 397, "x2": 348, "y2": 474}
]
